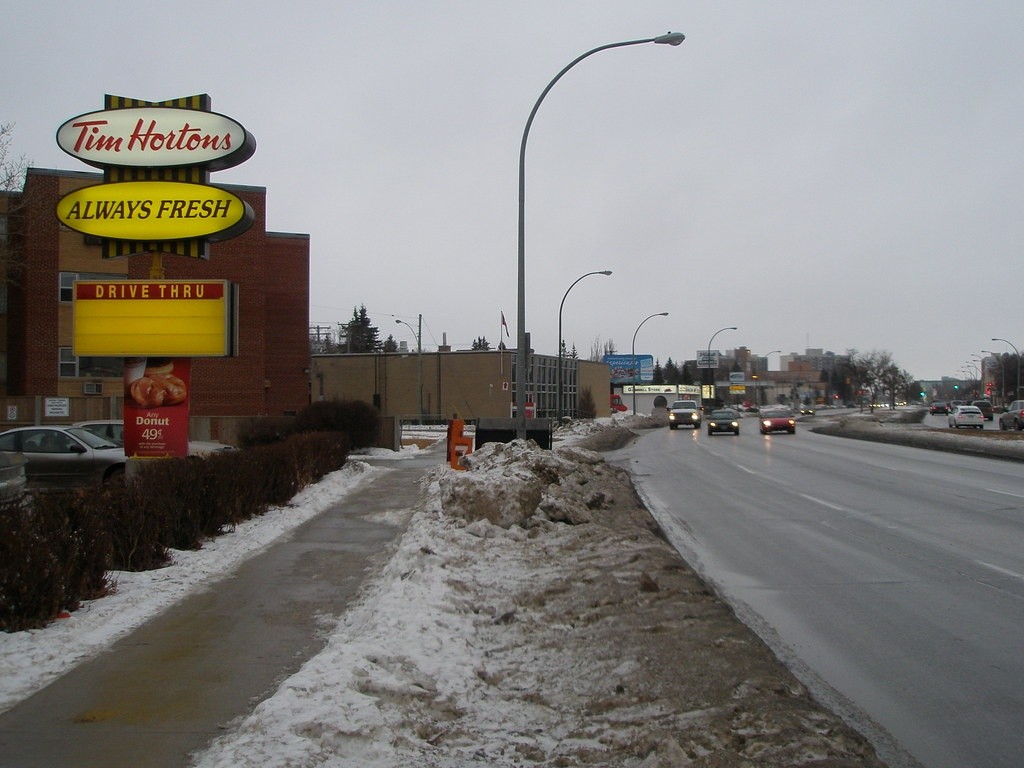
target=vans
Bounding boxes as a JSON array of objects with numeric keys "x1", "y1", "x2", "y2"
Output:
[{"x1": 610, "y1": 393, "x2": 628, "y2": 414}]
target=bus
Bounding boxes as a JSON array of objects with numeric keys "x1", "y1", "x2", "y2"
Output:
[{"x1": 800, "y1": 407, "x2": 816, "y2": 415}]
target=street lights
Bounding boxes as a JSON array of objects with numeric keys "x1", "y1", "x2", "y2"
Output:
[
  {"x1": 991, "y1": 338, "x2": 1021, "y2": 400},
  {"x1": 510, "y1": 30, "x2": 691, "y2": 455},
  {"x1": 395, "y1": 319, "x2": 422, "y2": 425},
  {"x1": 954, "y1": 353, "x2": 989, "y2": 399},
  {"x1": 633, "y1": 312, "x2": 668, "y2": 416},
  {"x1": 557, "y1": 269, "x2": 613, "y2": 430},
  {"x1": 981, "y1": 350, "x2": 1005, "y2": 403},
  {"x1": 754, "y1": 350, "x2": 782, "y2": 407},
  {"x1": 708, "y1": 327, "x2": 738, "y2": 416}
]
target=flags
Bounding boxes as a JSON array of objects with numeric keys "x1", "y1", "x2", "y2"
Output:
[{"x1": 502, "y1": 314, "x2": 509, "y2": 336}]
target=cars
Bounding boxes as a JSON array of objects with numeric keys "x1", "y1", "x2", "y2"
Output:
[
  {"x1": 760, "y1": 408, "x2": 797, "y2": 434},
  {"x1": 21, "y1": 419, "x2": 247, "y2": 467},
  {"x1": 1, "y1": 426, "x2": 130, "y2": 491},
  {"x1": 948, "y1": 405, "x2": 984, "y2": 430},
  {"x1": 928, "y1": 398, "x2": 974, "y2": 416},
  {"x1": 705, "y1": 410, "x2": 742, "y2": 436},
  {"x1": 998, "y1": 400, "x2": 1024, "y2": 430},
  {"x1": 0, "y1": 452, "x2": 31, "y2": 514}
]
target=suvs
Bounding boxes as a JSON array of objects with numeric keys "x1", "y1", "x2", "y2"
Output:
[
  {"x1": 666, "y1": 400, "x2": 702, "y2": 430},
  {"x1": 971, "y1": 400, "x2": 995, "y2": 422}
]
